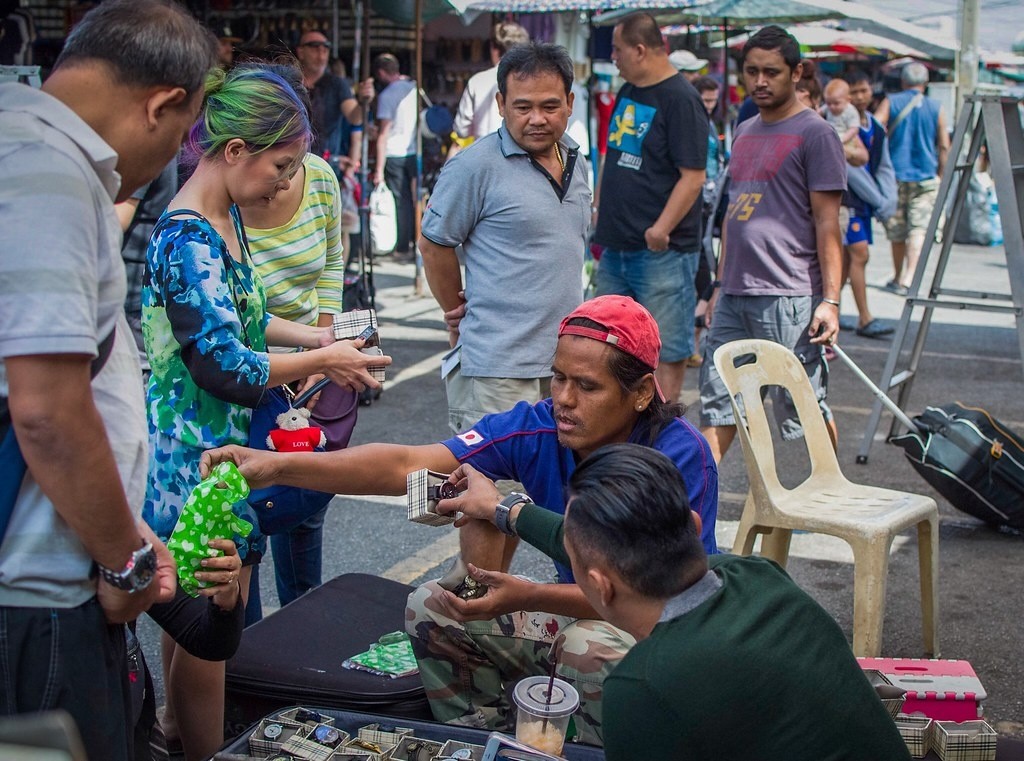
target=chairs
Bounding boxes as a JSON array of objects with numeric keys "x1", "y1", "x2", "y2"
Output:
[{"x1": 712, "y1": 336, "x2": 943, "y2": 660}]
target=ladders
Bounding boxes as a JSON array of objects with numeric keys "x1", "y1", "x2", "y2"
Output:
[{"x1": 855, "y1": 95, "x2": 1024, "y2": 465}]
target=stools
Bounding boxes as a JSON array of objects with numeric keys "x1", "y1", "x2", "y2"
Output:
[{"x1": 855, "y1": 657, "x2": 988, "y2": 722}]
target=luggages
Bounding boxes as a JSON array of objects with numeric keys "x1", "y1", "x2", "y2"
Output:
[
  {"x1": 296, "y1": 309, "x2": 386, "y2": 407},
  {"x1": 816, "y1": 334, "x2": 1024, "y2": 530}
]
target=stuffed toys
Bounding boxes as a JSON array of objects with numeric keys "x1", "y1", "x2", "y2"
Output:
[{"x1": 265, "y1": 407, "x2": 327, "y2": 452}]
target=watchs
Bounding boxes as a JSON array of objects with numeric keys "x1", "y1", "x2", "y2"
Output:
[
  {"x1": 440, "y1": 748, "x2": 474, "y2": 761},
  {"x1": 295, "y1": 710, "x2": 321, "y2": 723},
  {"x1": 268, "y1": 753, "x2": 295, "y2": 761},
  {"x1": 95, "y1": 538, "x2": 158, "y2": 594},
  {"x1": 314, "y1": 725, "x2": 342, "y2": 748},
  {"x1": 406, "y1": 739, "x2": 423, "y2": 761},
  {"x1": 263, "y1": 723, "x2": 284, "y2": 742},
  {"x1": 453, "y1": 574, "x2": 477, "y2": 596},
  {"x1": 427, "y1": 480, "x2": 458, "y2": 502},
  {"x1": 495, "y1": 491, "x2": 536, "y2": 538}
]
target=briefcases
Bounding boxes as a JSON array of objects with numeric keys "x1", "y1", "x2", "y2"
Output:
[{"x1": 223, "y1": 573, "x2": 437, "y2": 740}]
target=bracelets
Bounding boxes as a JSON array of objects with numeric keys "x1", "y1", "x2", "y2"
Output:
[
  {"x1": 713, "y1": 281, "x2": 721, "y2": 287},
  {"x1": 591, "y1": 207, "x2": 597, "y2": 213},
  {"x1": 823, "y1": 298, "x2": 840, "y2": 307}
]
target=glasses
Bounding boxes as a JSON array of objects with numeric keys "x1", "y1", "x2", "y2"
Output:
[
  {"x1": 798, "y1": 88, "x2": 818, "y2": 111},
  {"x1": 302, "y1": 40, "x2": 332, "y2": 48}
]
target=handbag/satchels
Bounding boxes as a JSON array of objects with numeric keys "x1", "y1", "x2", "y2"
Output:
[
  {"x1": 245, "y1": 386, "x2": 336, "y2": 536},
  {"x1": 412, "y1": 80, "x2": 454, "y2": 140},
  {"x1": 368, "y1": 182, "x2": 398, "y2": 256},
  {"x1": 308, "y1": 384, "x2": 358, "y2": 452}
]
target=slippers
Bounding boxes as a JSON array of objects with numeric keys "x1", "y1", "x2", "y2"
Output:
[
  {"x1": 839, "y1": 318, "x2": 856, "y2": 331},
  {"x1": 856, "y1": 319, "x2": 894, "y2": 335}
]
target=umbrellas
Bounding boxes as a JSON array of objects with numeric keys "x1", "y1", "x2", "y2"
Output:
[{"x1": 464, "y1": 0, "x2": 931, "y2": 117}]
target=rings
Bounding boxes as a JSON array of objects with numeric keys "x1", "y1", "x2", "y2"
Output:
[{"x1": 229, "y1": 572, "x2": 234, "y2": 583}]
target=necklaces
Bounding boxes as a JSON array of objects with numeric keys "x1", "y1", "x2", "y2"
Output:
[{"x1": 554, "y1": 143, "x2": 564, "y2": 169}]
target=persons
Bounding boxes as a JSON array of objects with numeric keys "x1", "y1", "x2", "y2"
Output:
[
  {"x1": 417, "y1": 11, "x2": 952, "y2": 572},
  {"x1": 197, "y1": 294, "x2": 725, "y2": 750},
  {"x1": 0, "y1": 0, "x2": 422, "y2": 761},
  {"x1": 434, "y1": 443, "x2": 912, "y2": 761}
]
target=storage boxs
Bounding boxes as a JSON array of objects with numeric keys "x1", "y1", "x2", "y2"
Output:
[
  {"x1": 406, "y1": 467, "x2": 457, "y2": 528},
  {"x1": 333, "y1": 308, "x2": 381, "y2": 348},
  {"x1": 305, "y1": 723, "x2": 351, "y2": 752},
  {"x1": 248, "y1": 717, "x2": 305, "y2": 758},
  {"x1": 932, "y1": 719, "x2": 997, "y2": 761},
  {"x1": 277, "y1": 707, "x2": 336, "y2": 739},
  {"x1": 367, "y1": 350, "x2": 385, "y2": 383},
  {"x1": 210, "y1": 707, "x2": 605, "y2": 761},
  {"x1": 340, "y1": 737, "x2": 396, "y2": 761},
  {"x1": 326, "y1": 752, "x2": 375, "y2": 761},
  {"x1": 357, "y1": 723, "x2": 414, "y2": 746},
  {"x1": 280, "y1": 735, "x2": 334, "y2": 761},
  {"x1": 893, "y1": 715, "x2": 933, "y2": 759},
  {"x1": 862, "y1": 669, "x2": 906, "y2": 720},
  {"x1": 436, "y1": 739, "x2": 486, "y2": 761},
  {"x1": 387, "y1": 735, "x2": 445, "y2": 761}
]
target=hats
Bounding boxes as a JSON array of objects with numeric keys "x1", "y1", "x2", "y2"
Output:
[
  {"x1": 667, "y1": 50, "x2": 710, "y2": 71},
  {"x1": 558, "y1": 295, "x2": 668, "y2": 404},
  {"x1": 212, "y1": 19, "x2": 245, "y2": 42}
]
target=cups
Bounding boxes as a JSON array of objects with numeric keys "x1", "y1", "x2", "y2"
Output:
[{"x1": 512, "y1": 676, "x2": 580, "y2": 757}]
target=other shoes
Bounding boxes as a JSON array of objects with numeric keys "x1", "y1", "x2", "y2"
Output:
[
  {"x1": 887, "y1": 282, "x2": 909, "y2": 296},
  {"x1": 687, "y1": 353, "x2": 703, "y2": 367}
]
